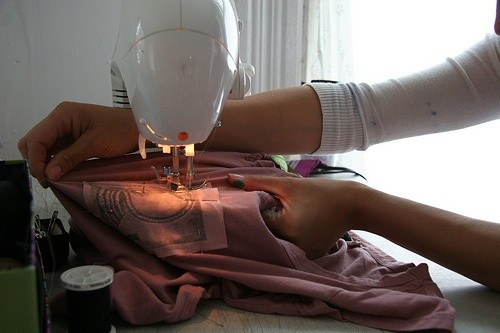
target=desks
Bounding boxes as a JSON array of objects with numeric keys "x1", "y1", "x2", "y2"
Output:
[{"x1": 40, "y1": 228, "x2": 500, "y2": 333}]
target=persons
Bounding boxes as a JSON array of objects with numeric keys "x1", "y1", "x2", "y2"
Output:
[{"x1": 19, "y1": 36, "x2": 500, "y2": 292}]
